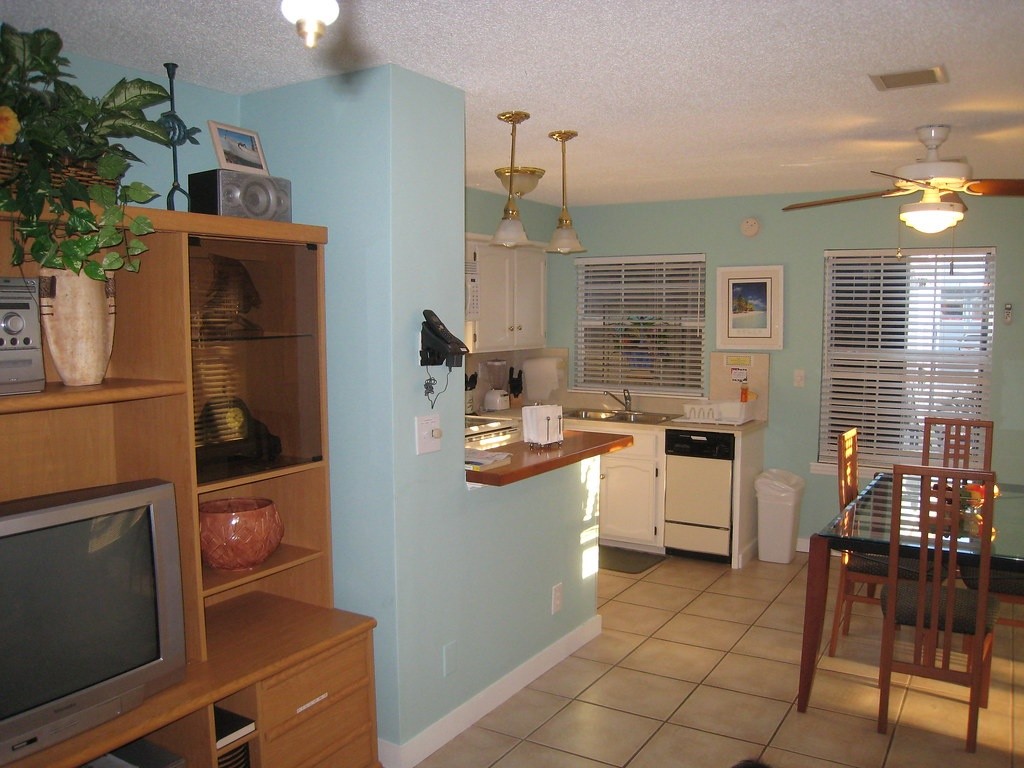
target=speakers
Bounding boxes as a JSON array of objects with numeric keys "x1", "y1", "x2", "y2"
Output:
[{"x1": 187, "y1": 169, "x2": 292, "y2": 224}]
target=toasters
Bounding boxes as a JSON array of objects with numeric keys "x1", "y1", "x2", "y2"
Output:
[{"x1": 522, "y1": 404, "x2": 564, "y2": 449}]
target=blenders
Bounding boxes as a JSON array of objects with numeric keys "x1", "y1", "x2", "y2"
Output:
[{"x1": 483, "y1": 359, "x2": 510, "y2": 410}]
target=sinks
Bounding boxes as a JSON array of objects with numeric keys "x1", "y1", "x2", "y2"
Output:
[
  {"x1": 562, "y1": 408, "x2": 620, "y2": 421},
  {"x1": 609, "y1": 412, "x2": 682, "y2": 425}
]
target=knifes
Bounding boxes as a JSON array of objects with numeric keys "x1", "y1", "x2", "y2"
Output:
[{"x1": 509, "y1": 367, "x2": 523, "y2": 395}]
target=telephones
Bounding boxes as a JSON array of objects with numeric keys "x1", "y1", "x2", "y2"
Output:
[{"x1": 421, "y1": 309, "x2": 469, "y2": 355}]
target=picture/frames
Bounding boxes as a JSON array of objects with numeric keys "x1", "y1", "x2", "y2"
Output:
[
  {"x1": 204, "y1": 120, "x2": 268, "y2": 177},
  {"x1": 715, "y1": 266, "x2": 783, "y2": 350}
]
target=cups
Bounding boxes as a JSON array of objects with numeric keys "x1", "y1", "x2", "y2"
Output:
[{"x1": 465, "y1": 390, "x2": 474, "y2": 415}]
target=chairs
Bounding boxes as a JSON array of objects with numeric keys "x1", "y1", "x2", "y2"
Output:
[
  {"x1": 827, "y1": 428, "x2": 948, "y2": 661},
  {"x1": 875, "y1": 417, "x2": 1024, "y2": 751}
]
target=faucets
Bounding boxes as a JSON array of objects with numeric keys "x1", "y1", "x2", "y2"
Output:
[{"x1": 603, "y1": 388, "x2": 632, "y2": 412}]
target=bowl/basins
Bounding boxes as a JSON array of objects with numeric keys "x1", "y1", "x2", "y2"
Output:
[{"x1": 198, "y1": 498, "x2": 284, "y2": 572}]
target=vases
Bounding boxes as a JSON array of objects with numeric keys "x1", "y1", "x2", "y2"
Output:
[
  {"x1": 40, "y1": 266, "x2": 116, "y2": 386},
  {"x1": 196, "y1": 498, "x2": 285, "y2": 573}
]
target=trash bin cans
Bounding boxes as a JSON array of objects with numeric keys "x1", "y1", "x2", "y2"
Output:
[{"x1": 755, "y1": 469, "x2": 805, "y2": 563}]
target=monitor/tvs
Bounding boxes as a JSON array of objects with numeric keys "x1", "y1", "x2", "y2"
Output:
[{"x1": 0, "y1": 477, "x2": 188, "y2": 765}]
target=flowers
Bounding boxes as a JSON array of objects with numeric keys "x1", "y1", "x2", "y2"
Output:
[{"x1": 1, "y1": 24, "x2": 171, "y2": 282}]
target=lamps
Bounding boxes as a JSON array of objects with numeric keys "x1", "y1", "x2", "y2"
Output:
[
  {"x1": 490, "y1": 111, "x2": 532, "y2": 247},
  {"x1": 280, "y1": 0, "x2": 340, "y2": 47},
  {"x1": 897, "y1": 186, "x2": 965, "y2": 233},
  {"x1": 494, "y1": 166, "x2": 543, "y2": 198},
  {"x1": 544, "y1": 129, "x2": 584, "y2": 252}
]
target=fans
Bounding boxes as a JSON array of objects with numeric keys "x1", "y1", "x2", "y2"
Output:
[{"x1": 780, "y1": 128, "x2": 1023, "y2": 210}]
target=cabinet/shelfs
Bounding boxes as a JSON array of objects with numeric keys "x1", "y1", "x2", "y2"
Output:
[
  {"x1": 0, "y1": 194, "x2": 382, "y2": 767},
  {"x1": 465, "y1": 233, "x2": 546, "y2": 353},
  {"x1": 564, "y1": 426, "x2": 666, "y2": 558}
]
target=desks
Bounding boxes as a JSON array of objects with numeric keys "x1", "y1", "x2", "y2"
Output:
[{"x1": 796, "y1": 468, "x2": 1023, "y2": 715}]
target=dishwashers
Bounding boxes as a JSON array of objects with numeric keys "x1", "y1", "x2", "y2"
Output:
[{"x1": 665, "y1": 429, "x2": 733, "y2": 557}]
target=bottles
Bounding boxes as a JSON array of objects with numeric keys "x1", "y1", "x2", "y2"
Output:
[{"x1": 741, "y1": 379, "x2": 748, "y2": 402}]
